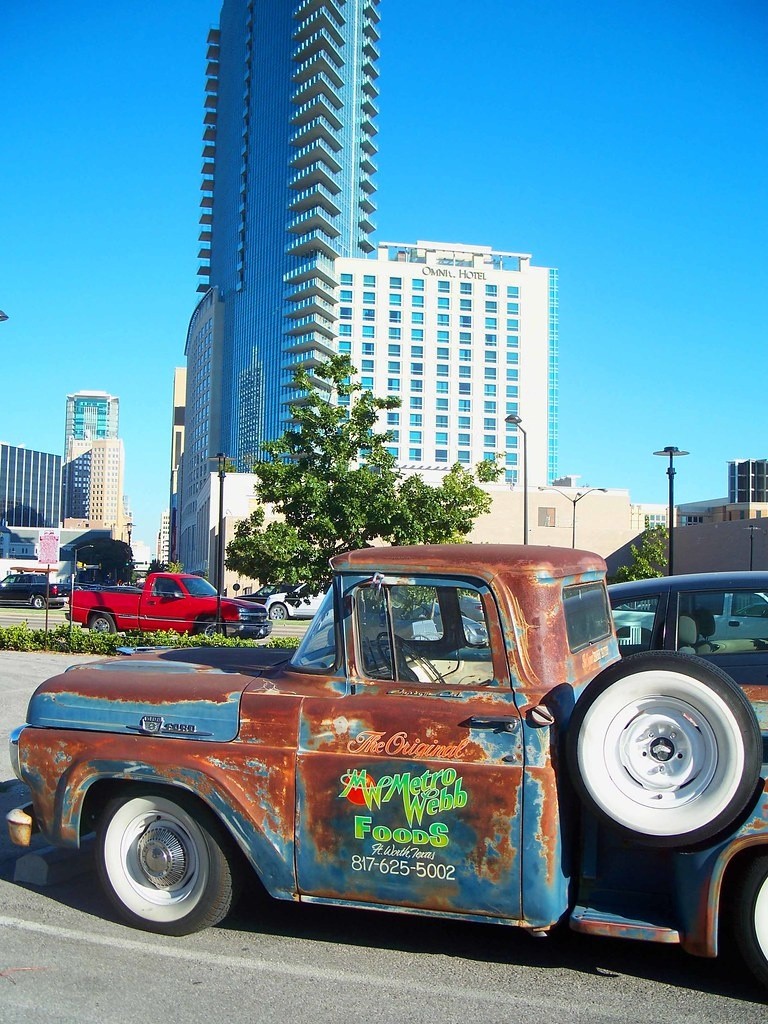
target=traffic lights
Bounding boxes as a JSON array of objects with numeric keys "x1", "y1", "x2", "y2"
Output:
[
  {"x1": 149, "y1": 567, "x2": 152, "y2": 571},
  {"x1": 124, "y1": 562, "x2": 129, "y2": 570}
]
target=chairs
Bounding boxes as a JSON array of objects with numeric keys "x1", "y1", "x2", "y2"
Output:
[{"x1": 679, "y1": 607, "x2": 715, "y2": 653}]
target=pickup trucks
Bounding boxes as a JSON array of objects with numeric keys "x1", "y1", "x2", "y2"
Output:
[
  {"x1": 65, "y1": 573, "x2": 273, "y2": 640},
  {"x1": 6, "y1": 545, "x2": 768, "y2": 1002},
  {"x1": 0, "y1": 574, "x2": 72, "y2": 610}
]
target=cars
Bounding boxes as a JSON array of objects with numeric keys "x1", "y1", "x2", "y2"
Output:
[
  {"x1": 74, "y1": 582, "x2": 143, "y2": 593},
  {"x1": 235, "y1": 582, "x2": 327, "y2": 620},
  {"x1": 405, "y1": 571, "x2": 768, "y2": 685}
]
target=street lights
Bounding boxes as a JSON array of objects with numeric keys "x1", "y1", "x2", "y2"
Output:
[
  {"x1": 653, "y1": 446, "x2": 690, "y2": 576},
  {"x1": 215, "y1": 452, "x2": 227, "y2": 634},
  {"x1": 123, "y1": 523, "x2": 136, "y2": 586},
  {"x1": 744, "y1": 525, "x2": 761, "y2": 571},
  {"x1": 70, "y1": 545, "x2": 93, "y2": 575},
  {"x1": 538, "y1": 486, "x2": 608, "y2": 549},
  {"x1": 505, "y1": 415, "x2": 529, "y2": 544}
]
